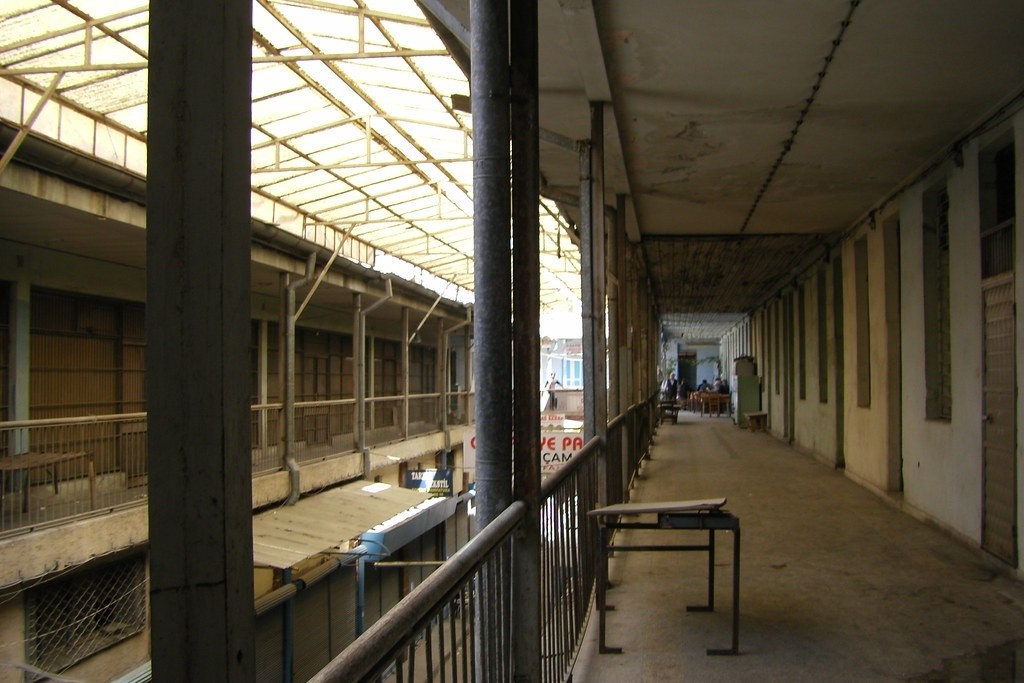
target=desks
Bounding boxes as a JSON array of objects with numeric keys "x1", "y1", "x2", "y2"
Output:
[
  {"x1": 689, "y1": 392, "x2": 731, "y2": 415},
  {"x1": 0, "y1": 448, "x2": 99, "y2": 517},
  {"x1": 657, "y1": 404, "x2": 681, "y2": 424}
]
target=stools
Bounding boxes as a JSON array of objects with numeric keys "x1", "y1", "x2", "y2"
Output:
[{"x1": 743, "y1": 412, "x2": 767, "y2": 430}]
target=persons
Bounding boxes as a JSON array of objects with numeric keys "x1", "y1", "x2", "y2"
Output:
[
  {"x1": 663, "y1": 373, "x2": 678, "y2": 402},
  {"x1": 544, "y1": 372, "x2": 564, "y2": 411},
  {"x1": 695, "y1": 377, "x2": 728, "y2": 413},
  {"x1": 679, "y1": 379, "x2": 688, "y2": 410}
]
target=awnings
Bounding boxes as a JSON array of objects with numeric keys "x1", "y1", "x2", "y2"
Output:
[{"x1": 251, "y1": 482, "x2": 435, "y2": 570}]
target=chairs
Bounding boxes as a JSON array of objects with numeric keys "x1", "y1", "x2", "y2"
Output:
[
  {"x1": 694, "y1": 392, "x2": 721, "y2": 417},
  {"x1": 659, "y1": 401, "x2": 675, "y2": 425}
]
